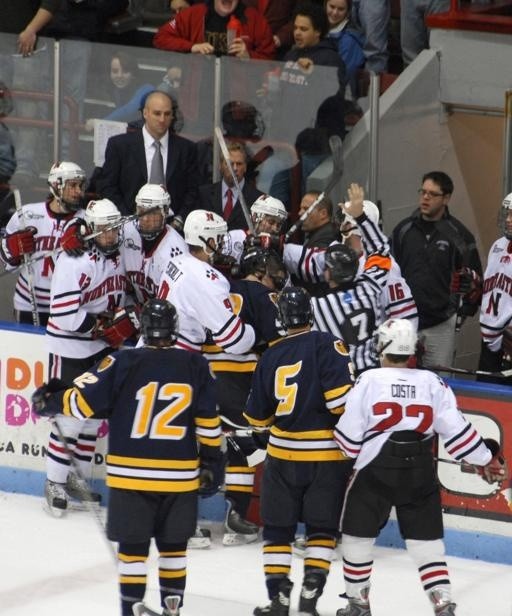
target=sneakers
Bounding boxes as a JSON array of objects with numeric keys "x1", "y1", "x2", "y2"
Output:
[
  {"x1": 223, "y1": 497, "x2": 259, "y2": 534},
  {"x1": 294, "y1": 535, "x2": 338, "y2": 551},
  {"x1": 193, "y1": 525, "x2": 211, "y2": 537},
  {"x1": 44, "y1": 474, "x2": 101, "y2": 509},
  {"x1": 254, "y1": 573, "x2": 371, "y2": 616},
  {"x1": 429, "y1": 589, "x2": 456, "y2": 616}
]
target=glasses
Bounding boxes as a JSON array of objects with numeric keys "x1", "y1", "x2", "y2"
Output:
[{"x1": 418, "y1": 189, "x2": 445, "y2": 197}]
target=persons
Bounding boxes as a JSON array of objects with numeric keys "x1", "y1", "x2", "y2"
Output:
[
  {"x1": 476, "y1": 193, "x2": 512, "y2": 385},
  {"x1": 386, "y1": 171, "x2": 483, "y2": 381},
  {"x1": 0, "y1": 0, "x2": 452, "y2": 202},
  {"x1": 243, "y1": 286, "x2": 355, "y2": 616},
  {"x1": 330, "y1": 318, "x2": 506, "y2": 616},
  {"x1": 0, "y1": 91, "x2": 419, "y2": 547},
  {"x1": 32, "y1": 296, "x2": 227, "y2": 615}
]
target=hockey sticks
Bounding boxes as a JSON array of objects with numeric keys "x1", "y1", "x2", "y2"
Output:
[
  {"x1": 283, "y1": 136, "x2": 345, "y2": 238},
  {"x1": 246, "y1": 144, "x2": 276, "y2": 173},
  {"x1": 0, "y1": 205, "x2": 160, "y2": 276},
  {"x1": 226, "y1": 433, "x2": 267, "y2": 468},
  {"x1": 214, "y1": 126, "x2": 256, "y2": 234}
]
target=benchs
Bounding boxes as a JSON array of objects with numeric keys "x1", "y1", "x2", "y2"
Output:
[{"x1": 89, "y1": 0, "x2": 421, "y2": 198}]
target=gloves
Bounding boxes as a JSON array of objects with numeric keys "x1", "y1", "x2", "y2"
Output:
[
  {"x1": 450, "y1": 268, "x2": 481, "y2": 305},
  {"x1": 91, "y1": 305, "x2": 143, "y2": 349},
  {"x1": 198, "y1": 458, "x2": 225, "y2": 498},
  {"x1": 32, "y1": 378, "x2": 66, "y2": 417},
  {"x1": 477, "y1": 439, "x2": 507, "y2": 482},
  {"x1": 59, "y1": 224, "x2": 87, "y2": 256},
  {"x1": 243, "y1": 233, "x2": 284, "y2": 254},
  {"x1": 6, "y1": 228, "x2": 36, "y2": 258}
]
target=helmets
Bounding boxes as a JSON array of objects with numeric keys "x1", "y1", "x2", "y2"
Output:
[
  {"x1": 371, "y1": 318, "x2": 416, "y2": 356},
  {"x1": 277, "y1": 284, "x2": 314, "y2": 331},
  {"x1": 47, "y1": 160, "x2": 171, "y2": 256},
  {"x1": 183, "y1": 194, "x2": 289, "y2": 291},
  {"x1": 140, "y1": 299, "x2": 180, "y2": 347},
  {"x1": 498, "y1": 192, "x2": 512, "y2": 240},
  {"x1": 316, "y1": 241, "x2": 360, "y2": 284},
  {"x1": 334, "y1": 200, "x2": 380, "y2": 239}
]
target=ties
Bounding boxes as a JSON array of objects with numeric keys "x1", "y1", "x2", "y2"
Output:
[
  {"x1": 150, "y1": 140, "x2": 162, "y2": 184},
  {"x1": 223, "y1": 189, "x2": 232, "y2": 219}
]
